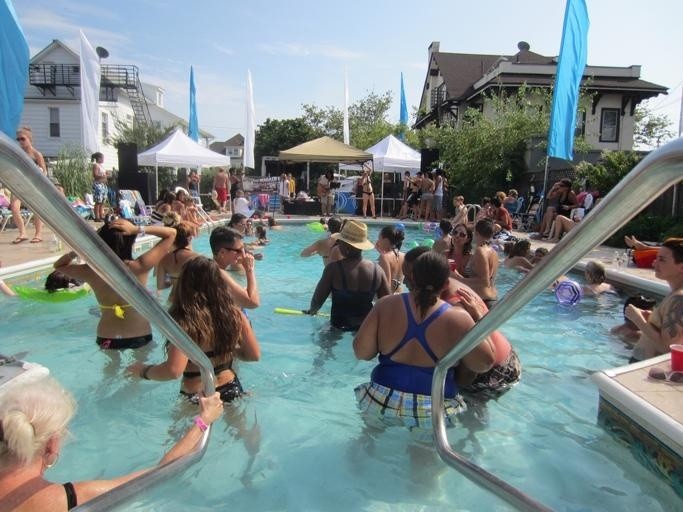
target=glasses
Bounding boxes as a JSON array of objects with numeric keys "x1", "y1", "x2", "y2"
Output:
[
  {"x1": 452, "y1": 230, "x2": 468, "y2": 238},
  {"x1": 247, "y1": 223, "x2": 252, "y2": 225},
  {"x1": 15, "y1": 137, "x2": 27, "y2": 141},
  {"x1": 220, "y1": 244, "x2": 245, "y2": 255},
  {"x1": 558, "y1": 183, "x2": 568, "y2": 187},
  {"x1": 237, "y1": 223, "x2": 246, "y2": 228}
]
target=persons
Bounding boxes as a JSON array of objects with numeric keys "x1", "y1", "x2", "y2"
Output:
[
  {"x1": 301, "y1": 218, "x2": 392, "y2": 333},
  {"x1": 147, "y1": 188, "x2": 181, "y2": 227},
  {"x1": 185, "y1": 169, "x2": 199, "y2": 196},
  {"x1": 431, "y1": 220, "x2": 453, "y2": 260},
  {"x1": 232, "y1": 188, "x2": 255, "y2": 218},
  {"x1": 503, "y1": 188, "x2": 522, "y2": 218},
  {"x1": 361, "y1": 169, "x2": 376, "y2": 217},
  {"x1": 431, "y1": 168, "x2": 444, "y2": 221},
  {"x1": 459, "y1": 196, "x2": 468, "y2": 214},
  {"x1": 490, "y1": 196, "x2": 512, "y2": 235},
  {"x1": 400, "y1": 172, "x2": 424, "y2": 220},
  {"x1": 127, "y1": 253, "x2": 261, "y2": 441},
  {"x1": 89, "y1": 150, "x2": 108, "y2": 222},
  {"x1": 476, "y1": 196, "x2": 494, "y2": 223},
  {"x1": 317, "y1": 217, "x2": 329, "y2": 230},
  {"x1": 560, "y1": 261, "x2": 619, "y2": 300},
  {"x1": 260, "y1": 216, "x2": 282, "y2": 231},
  {"x1": 185, "y1": 194, "x2": 200, "y2": 226},
  {"x1": 228, "y1": 166, "x2": 243, "y2": 203},
  {"x1": 451, "y1": 196, "x2": 465, "y2": 225},
  {"x1": 280, "y1": 173, "x2": 290, "y2": 213},
  {"x1": 0, "y1": 275, "x2": 15, "y2": 296},
  {"x1": 351, "y1": 252, "x2": 497, "y2": 498},
  {"x1": 608, "y1": 294, "x2": 656, "y2": 342},
  {"x1": 315, "y1": 167, "x2": 335, "y2": 217},
  {"x1": 446, "y1": 223, "x2": 473, "y2": 277},
  {"x1": 453, "y1": 216, "x2": 498, "y2": 309},
  {"x1": 251, "y1": 225, "x2": 272, "y2": 247},
  {"x1": 442, "y1": 170, "x2": 449, "y2": 218},
  {"x1": 400, "y1": 244, "x2": 522, "y2": 432},
  {"x1": 44, "y1": 268, "x2": 84, "y2": 295},
  {"x1": 517, "y1": 247, "x2": 549, "y2": 273},
  {"x1": 300, "y1": 215, "x2": 343, "y2": 268},
  {"x1": 499, "y1": 239, "x2": 535, "y2": 268},
  {"x1": 547, "y1": 193, "x2": 599, "y2": 243},
  {"x1": 375, "y1": 222, "x2": 407, "y2": 296},
  {"x1": 52, "y1": 217, "x2": 178, "y2": 375},
  {"x1": 245, "y1": 219, "x2": 254, "y2": 238},
  {"x1": 228, "y1": 211, "x2": 264, "y2": 272},
  {"x1": 624, "y1": 231, "x2": 683, "y2": 251},
  {"x1": 495, "y1": 190, "x2": 507, "y2": 209},
  {"x1": 403, "y1": 171, "x2": 411, "y2": 204},
  {"x1": 287, "y1": 173, "x2": 296, "y2": 198},
  {"x1": 212, "y1": 166, "x2": 230, "y2": 212},
  {"x1": 171, "y1": 184, "x2": 186, "y2": 213},
  {"x1": 153, "y1": 211, "x2": 184, "y2": 276},
  {"x1": 156, "y1": 220, "x2": 200, "y2": 290},
  {"x1": 417, "y1": 173, "x2": 435, "y2": 222},
  {"x1": 532, "y1": 177, "x2": 579, "y2": 241},
  {"x1": 1, "y1": 351, "x2": 223, "y2": 512},
  {"x1": 9, "y1": 126, "x2": 49, "y2": 244},
  {"x1": 503, "y1": 242, "x2": 513, "y2": 256},
  {"x1": 622, "y1": 237, "x2": 683, "y2": 367},
  {"x1": 209, "y1": 225, "x2": 261, "y2": 311}
]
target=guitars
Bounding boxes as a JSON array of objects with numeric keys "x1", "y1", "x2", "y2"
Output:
[{"x1": 315, "y1": 182, "x2": 341, "y2": 197}]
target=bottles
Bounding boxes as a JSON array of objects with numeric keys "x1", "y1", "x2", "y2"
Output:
[
  {"x1": 623, "y1": 253, "x2": 629, "y2": 270},
  {"x1": 50, "y1": 231, "x2": 57, "y2": 249},
  {"x1": 615, "y1": 250, "x2": 621, "y2": 270},
  {"x1": 56, "y1": 236, "x2": 62, "y2": 250}
]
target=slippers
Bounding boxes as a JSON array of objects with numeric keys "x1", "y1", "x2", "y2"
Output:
[
  {"x1": 11, "y1": 236, "x2": 29, "y2": 244},
  {"x1": 30, "y1": 237, "x2": 43, "y2": 242}
]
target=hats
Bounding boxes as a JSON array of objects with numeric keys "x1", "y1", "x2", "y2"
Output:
[
  {"x1": 174, "y1": 186, "x2": 190, "y2": 198},
  {"x1": 330, "y1": 219, "x2": 374, "y2": 251}
]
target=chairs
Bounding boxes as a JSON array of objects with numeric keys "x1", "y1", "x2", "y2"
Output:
[
  {"x1": 118, "y1": 189, "x2": 152, "y2": 220},
  {"x1": 512, "y1": 197, "x2": 541, "y2": 232},
  {"x1": 87, "y1": 193, "x2": 110, "y2": 218},
  {"x1": 175, "y1": 186, "x2": 203, "y2": 211},
  {"x1": 512, "y1": 197, "x2": 525, "y2": 223},
  {"x1": 0, "y1": 208, "x2": 33, "y2": 232}
]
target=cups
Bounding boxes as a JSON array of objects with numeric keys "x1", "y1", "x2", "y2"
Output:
[{"x1": 670, "y1": 344, "x2": 683, "y2": 371}]
